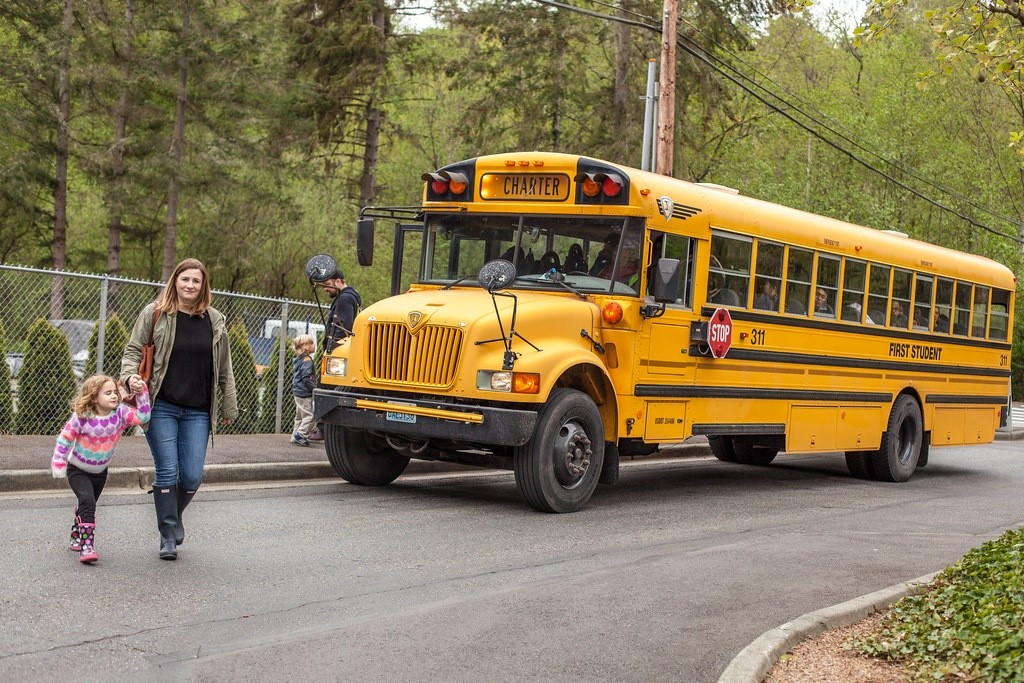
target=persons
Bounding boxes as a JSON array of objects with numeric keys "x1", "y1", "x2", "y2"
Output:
[
  {"x1": 843, "y1": 300, "x2": 875, "y2": 325},
  {"x1": 648, "y1": 235, "x2": 662, "y2": 296},
  {"x1": 309, "y1": 267, "x2": 361, "y2": 440},
  {"x1": 589, "y1": 234, "x2": 640, "y2": 293},
  {"x1": 933, "y1": 307, "x2": 951, "y2": 333},
  {"x1": 122, "y1": 258, "x2": 238, "y2": 560},
  {"x1": 51, "y1": 375, "x2": 151, "y2": 561},
  {"x1": 912, "y1": 306, "x2": 928, "y2": 326},
  {"x1": 890, "y1": 300, "x2": 908, "y2": 327},
  {"x1": 814, "y1": 287, "x2": 833, "y2": 314},
  {"x1": 291, "y1": 334, "x2": 316, "y2": 447},
  {"x1": 759, "y1": 274, "x2": 792, "y2": 313}
]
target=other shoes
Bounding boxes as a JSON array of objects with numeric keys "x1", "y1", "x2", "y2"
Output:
[{"x1": 310, "y1": 431, "x2": 324, "y2": 440}]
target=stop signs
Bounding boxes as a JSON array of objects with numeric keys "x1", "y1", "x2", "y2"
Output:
[{"x1": 706, "y1": 307, "x2": 731, "y2": 360}]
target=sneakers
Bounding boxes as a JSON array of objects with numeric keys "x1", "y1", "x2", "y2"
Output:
[{"x1": 293, "y1": 432, "x2": 310, "y2": 446}]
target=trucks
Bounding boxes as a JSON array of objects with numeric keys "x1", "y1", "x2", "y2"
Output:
[{"x1": 261, "y1": 318, "x2": 326, "y2": 363}]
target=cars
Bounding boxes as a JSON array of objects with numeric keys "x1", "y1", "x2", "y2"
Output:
[{"x1": 3, "y1": 319, "x2": 133, "y2": 416}]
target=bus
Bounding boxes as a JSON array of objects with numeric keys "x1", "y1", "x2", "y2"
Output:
[{"x1": 305, "y1": 150, "x2": 1018, "y2": 515}]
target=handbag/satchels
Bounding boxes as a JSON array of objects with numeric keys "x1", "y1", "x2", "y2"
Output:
[{"x1": 116, "y1": 300, "x2": 160, "y2": 406}]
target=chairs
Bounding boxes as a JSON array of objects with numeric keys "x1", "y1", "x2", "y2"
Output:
[{"x1": 711, "y1": 290, "x2": 1004, "y2": 338}]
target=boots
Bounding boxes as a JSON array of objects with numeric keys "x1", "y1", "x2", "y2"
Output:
[
  {"x1": 173, "y1": 476, "x2": 202, "y2": 545},
  {"x1": 70, "y1": 510, "x2": 82, "y2": 551},
  {"x1": 74, "y1": 516, "x2": 98, "y2": 562},
  {"x1": 147, "y1": 481, "x2": 178, "y2": 560}
]
